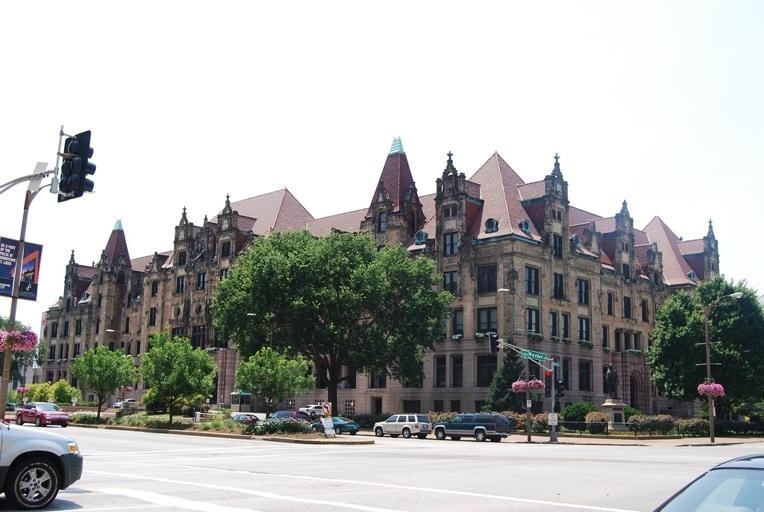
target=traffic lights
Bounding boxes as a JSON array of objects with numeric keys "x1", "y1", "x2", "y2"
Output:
[
  {"x1": 544, "y1": 370, "x2": 564, "y2": 398},
  {"x1": 57, "y1": 129, "x2": 96, "y2": 202},
  {"x1": 488, "y1": 332, "x2": 499, "y2": 353}
]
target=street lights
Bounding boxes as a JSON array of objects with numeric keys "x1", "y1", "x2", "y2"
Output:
[
  {"x1": 497, "y1": 288, "x2": 531, "y2": 442},
  {"x1": 704, "y1": 292, "x2": 745, "y2": 443},
  {"x1": 106, "y1": 329, "x2": 127, "y2": 409}
]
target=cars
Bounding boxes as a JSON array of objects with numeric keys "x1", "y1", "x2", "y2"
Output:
[
  {"x1": 231, "y1": 404, "x2": 360, "y2": 435},
  {"x1": 15, "y1": 402, "x2": 70, "y2": 427},
  {"x1": 434, "y1": 413, "x2": 511, "y2": 442},
  {"x1": 0, "y1": 419, "x2": 83, "y2": 510},
  {"x1": 650, "y1": 452, "x2": 764, "y2": 512},
  {"x1": 113, "y1": 399, "x2": 137, "y2": 408},
  {"x1": 373, "y1": 414, "x2": 432, "y2": 438}
]
